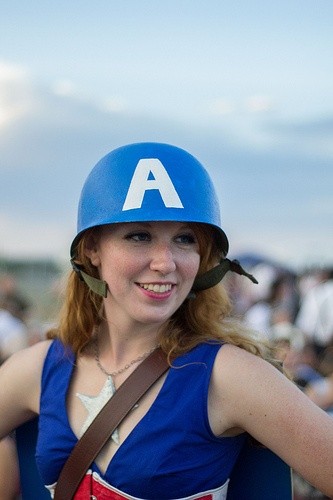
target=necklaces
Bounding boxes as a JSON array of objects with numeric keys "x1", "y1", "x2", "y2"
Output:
[{"x1": 75, "y1": 326, "x2": 161, "y2": 444}]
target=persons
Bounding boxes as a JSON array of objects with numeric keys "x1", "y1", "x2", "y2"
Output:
[
  {"x1": 0, "y1": 142, "x2": 333, "y2": 500},
  {"x1": 0, "y1": 261, "x2": 333, "y2": 500}
]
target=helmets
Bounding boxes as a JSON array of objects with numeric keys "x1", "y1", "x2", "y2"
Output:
[{"x1": 69, "y1": 141, "x2": 228, "y2": 263}]
